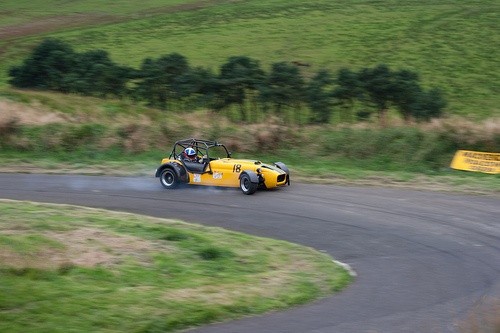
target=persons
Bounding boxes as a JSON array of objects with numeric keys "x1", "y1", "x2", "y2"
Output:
[{"x1": 183, "y1": 147, "x2": 207, "y2": 164}]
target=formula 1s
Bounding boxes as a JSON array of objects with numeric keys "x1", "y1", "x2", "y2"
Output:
[{"x1": 154, "y1": 139, "x2": 291, "y2": 195}]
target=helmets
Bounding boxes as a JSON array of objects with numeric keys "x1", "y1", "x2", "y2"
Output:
[{"x1": 184, "y1": 147, "x2": 196, "y2": 159}]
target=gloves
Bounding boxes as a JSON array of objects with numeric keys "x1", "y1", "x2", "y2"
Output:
[
  {"x1": 199, "y1": 158, "x2": 203, "y2": 163},
  {"x1": 203, "y1": 154, "x2": 207, "y2": 158}
]
